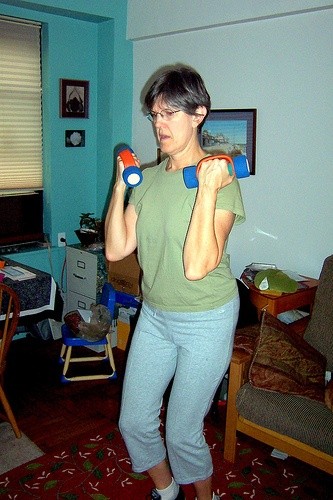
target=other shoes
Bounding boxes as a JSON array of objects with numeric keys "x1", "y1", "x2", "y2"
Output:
[{"x1": 151, "y1": 485, "x2": 185, "y2": 500}]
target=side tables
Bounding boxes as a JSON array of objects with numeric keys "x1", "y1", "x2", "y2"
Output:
[
  {"x1": 107, "y1": 252, "x2": 140, "y2": 296},
  {"x1": 241, "y1": 266, "x2": 319, "y2": 331}
]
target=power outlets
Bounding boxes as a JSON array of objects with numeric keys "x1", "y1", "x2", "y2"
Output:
[{"x1": 57, "y1": 233, "x2": 66, "y2": 247}]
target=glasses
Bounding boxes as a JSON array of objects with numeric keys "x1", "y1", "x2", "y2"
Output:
[{"x1": 145, "y1": 109, "x2": 185, "y2": 123}]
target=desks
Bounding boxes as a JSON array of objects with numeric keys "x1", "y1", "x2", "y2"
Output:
[{"x1": 0, "y1": 252, "x2": 57, "y2": 321}]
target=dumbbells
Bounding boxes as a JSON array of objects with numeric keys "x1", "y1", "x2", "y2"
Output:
[
  {"x1": 116, "y1": 145, "x2": 143, "y2": 187},
  {"x1": 182, "y1": 154, "x2": 250, "y2": 190}
]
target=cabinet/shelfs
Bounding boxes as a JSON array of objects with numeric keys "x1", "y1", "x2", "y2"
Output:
[{"x1": 66, "y1": 245, "x2": 106, "y2": 316}]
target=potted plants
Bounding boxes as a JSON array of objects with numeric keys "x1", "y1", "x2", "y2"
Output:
[{"x1": 74, "y1": 212, "x2": 100, "y2": 246}]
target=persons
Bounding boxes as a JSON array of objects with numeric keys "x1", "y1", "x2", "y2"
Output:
[{"x1": 105, "y1": 68, "x2": 246, "y2": 500}]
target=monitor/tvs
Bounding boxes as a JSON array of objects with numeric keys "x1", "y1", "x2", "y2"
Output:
[{"x1": 1, "y1": 190, "x2": 44, "y2": 253}]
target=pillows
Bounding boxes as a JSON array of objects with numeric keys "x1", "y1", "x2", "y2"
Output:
[{"x1": 248, "y1": 310, "x2": 327, "y2": 404}]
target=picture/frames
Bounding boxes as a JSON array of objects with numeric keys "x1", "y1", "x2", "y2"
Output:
[
  {"x1": 157, "y1": 109, "x2": 257, "y2": 176},
  {"x1": 64, "y1": 129, "x2": 86, "y2": 148},
  {"x1": 59, "y1": 79, "x2": 90, "y2": 119}
]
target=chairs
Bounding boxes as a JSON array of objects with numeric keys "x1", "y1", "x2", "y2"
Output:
[
  {"x1": 58, "y1": 282, "x2": 119, "y2": 384},
  {"x1": 223, "y1": 254, "x2": 333, "y2": 479},
  {"x1": 0, "y1": 282, "x2": 22, "y2": 439}
]
